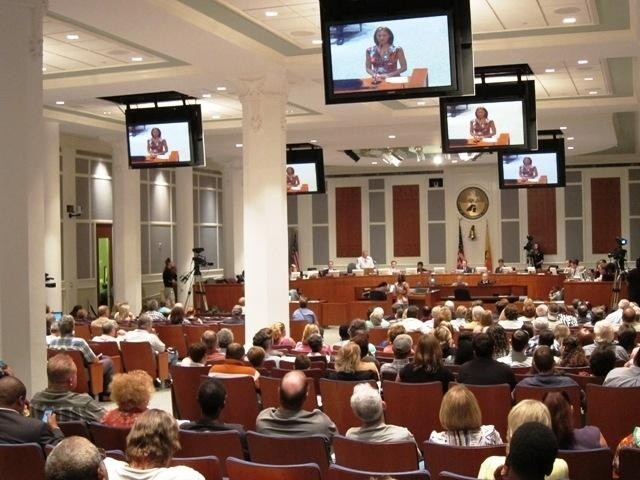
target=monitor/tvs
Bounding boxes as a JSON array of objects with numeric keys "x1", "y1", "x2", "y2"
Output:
[
  {"x1": 53, "y1": 312, "x2": 63, "y2": 322},
  {"x1": 125, "y1": 104, "x2": 205, "y2": 170},
  {"x1": 439, "y1": 79, "x2": 539, "y2": 153},
  {"x1": 287, "y1": 148, "x2": 326, "y2": 194},
  {"x1": 290, "y1": 266, "x2": 586, "y2": 282},
  {"x1": 199, "y1": 268, "x2": 225, "y2": 282},
  {"x1": 496, "y1": 135, "x2": 567, "y2": 189}
]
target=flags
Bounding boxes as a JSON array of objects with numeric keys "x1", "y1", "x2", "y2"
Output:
[
  {"x1": 484, "y1": 222, "x2": 492, "y2": 272},
  {"x1": 457, "y1": 220, "x2": 465, "y2": 269}
]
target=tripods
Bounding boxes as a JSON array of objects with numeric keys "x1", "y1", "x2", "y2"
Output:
[
  {"x1": 525, "y1": 253, "x2": 535, "y2": 267},
  {"x1": 184, "y1": 265, "x2": 209, "y2": 313},
  {"x1": 611, "y1": 270, "x2": 629, "y2": 309}
]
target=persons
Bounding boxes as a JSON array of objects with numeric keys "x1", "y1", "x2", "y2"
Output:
[
  {"x1": 364, "y1": 26, "x2": 407, "y2": 80},
  {"x1": 146, "y1": 127, "x2": 169, "y2": 157},
  {"x1": 470, "y1": 106, "x2": 496, "y2": 139},
  {"x1": 518, "y1": 156, "x2": 538, "y2": 180},
  {"x1": 0, "y1": 236, "x2": 640, "y2": 479},
  {"x1": 286, "y1": 167, "x2": 302, "y2": 188}
]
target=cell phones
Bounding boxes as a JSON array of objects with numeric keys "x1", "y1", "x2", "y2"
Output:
[{"x1": 41, "y1": 408, "x2": 53, "y2": 423}]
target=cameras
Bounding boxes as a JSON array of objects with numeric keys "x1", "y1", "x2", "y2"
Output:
[{"x1": 318, "y1": 0, "x2": 477, "y2": 105}]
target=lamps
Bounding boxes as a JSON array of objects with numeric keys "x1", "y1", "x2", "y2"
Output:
[
  {"x1": 382, "y1": 145, "x2": 467, "y2": 167},
  {"x1": 65, "y1": 202, "x2": 84, "y2": 219}
]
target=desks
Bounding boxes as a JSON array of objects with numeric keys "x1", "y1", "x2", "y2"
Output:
[
  {"x1": 194, "y1": 281, "x2": 245, "y2": 315},
  {"x1": 291, "y1": 270, "x2": 566, "y2": 299},
  {"x1": 563, "y1": 279, "x2": 630, "y2": 313}
]
